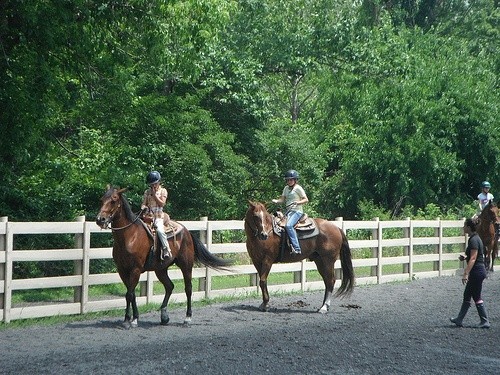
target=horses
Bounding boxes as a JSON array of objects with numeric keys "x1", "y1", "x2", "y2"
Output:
[
  {"x1": 242, "y1": 198, "x2": 358, "y2": 313},
  {"x1": 96, "y1": 183, "x2": 236, "y2": 329},
  {"x1": 471, "y1": 198, "x2": 500, "y2": 272}
]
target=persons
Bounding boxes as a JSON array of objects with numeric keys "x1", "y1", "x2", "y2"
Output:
[
  {"x1": 478, "y1": 181, "x2": 494, "y2": 214},
  {"x1": 449, "y1": 219, "x2": 490, "y2": 328},
  {"x1": 141, "y1": 171, "x2": 172, "y2": 258},
  {"x1": 272, "y1": 170, "x2": 309, "y2": 255}
]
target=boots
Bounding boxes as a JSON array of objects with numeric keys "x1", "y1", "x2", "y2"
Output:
[
  {"x1": 474, "y1": 303, "x2": 490, "y2": 327},
  {"x1": 450, "y1": 299, "x2": 471, "y2": 326}
]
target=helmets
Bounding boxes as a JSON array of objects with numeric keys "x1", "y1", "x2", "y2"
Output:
[
  {"x1": 146, "y1": 171, "x2": 161, "y2": 185},
  {"x1": 285, "y1": 170, "x2": 297, "y2": 179},
  {"x1": 480, "y1": 181, "x2": 491, "y2": 188}
]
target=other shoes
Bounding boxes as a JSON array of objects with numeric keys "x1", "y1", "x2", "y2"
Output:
[{"x1": 498, "y1": 236, "x2": 500, "y2": 242}]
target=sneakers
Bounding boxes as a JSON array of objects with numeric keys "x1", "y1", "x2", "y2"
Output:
[
  {"x1": 163, "y1": 249, "x2": 172, "y2": 258},
  {"x1": 290, "y1": 250, "x2": 301, "y2": 255}
]
